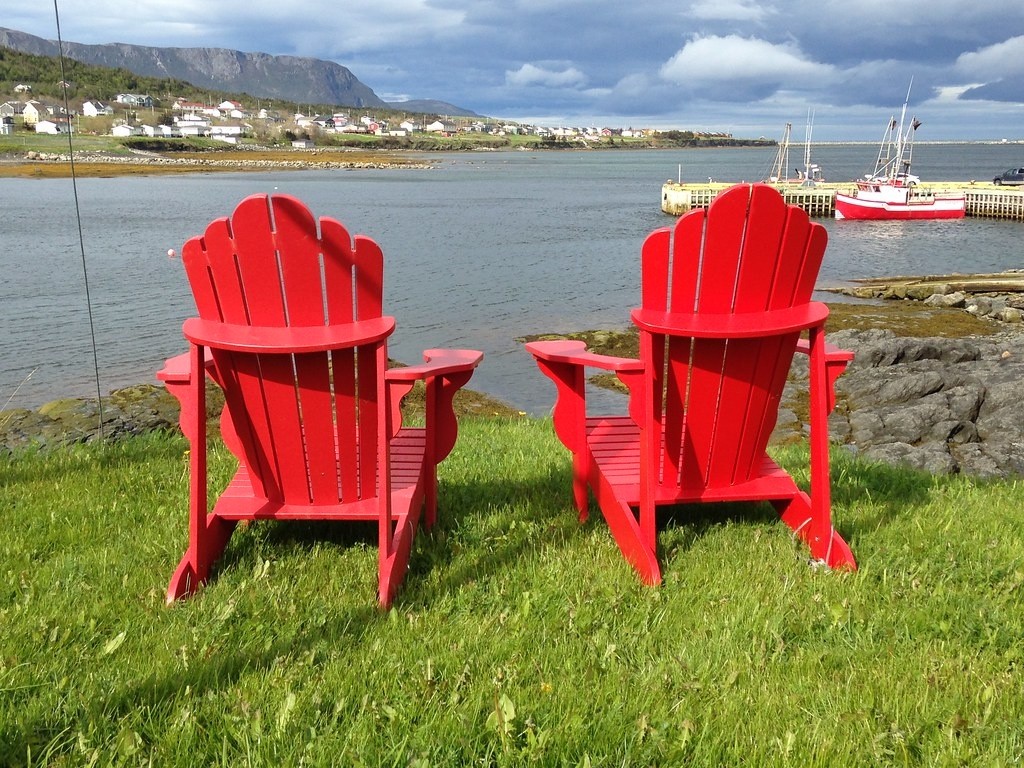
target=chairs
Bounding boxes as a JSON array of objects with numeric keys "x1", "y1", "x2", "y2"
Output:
[
  {"x1": 524, "y1": 181, "x2": 858, "y2": 584},
  {"x1": 154, "y1": 191, "x2": 483, "y2": 614}
]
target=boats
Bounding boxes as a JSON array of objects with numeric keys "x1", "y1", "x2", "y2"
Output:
[
  {"x1": 761, "y1": 163, "x2": 825, "y2": 183},
  {"x1": 834, "y1": 74, "x2": 967, "y2": 221}
]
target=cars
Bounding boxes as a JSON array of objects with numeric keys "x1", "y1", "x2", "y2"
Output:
[{"x1": 874, "y1": 172, "x2": 920, "y2": 186}]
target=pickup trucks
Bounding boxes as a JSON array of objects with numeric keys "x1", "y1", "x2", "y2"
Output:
[{"x1": 993, "y1": 168, "x2": 1024, "y2": 188}]
